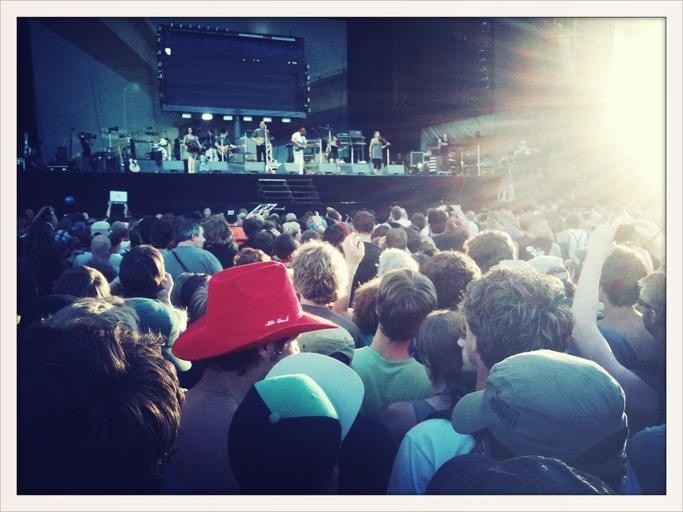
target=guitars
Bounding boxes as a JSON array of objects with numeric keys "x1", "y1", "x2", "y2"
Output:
[
  {"x1": 255, "y1": 137, "x2": 274, "y2": 146},
  {"x1": 218, "y1": 145, "x2": 241, "y2": 154},
  {"x1": 129, "y1": 143, "x2": 140, "y2": 172}
]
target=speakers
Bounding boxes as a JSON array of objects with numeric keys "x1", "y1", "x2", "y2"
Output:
[
  {"x1": 131, "y1": 139, "x2": 147, "y2": 160},
  {"x1": 384, "y1": 165, "x2": 404, "y2": 176},
  {"x1": 162, "y1": 161, "x2": 183, "y2": 173},
  {"x1": 343, "y1": 163, "x2": 371, "y2": 176},
  {"x1": 411, "y1": 152, "x2": 424, "y2": 172},
  {"x1": 316, "y1": 163, "x2": 336, "y2": 174},
  {"x1": 207, "y1": 161, "x2": 227, "y2": 173},
  {"x1": 244, "y1": 162, "x2": 264, "y2": 172},
  {"x1": 279, "y1": 163, "x2": 298, "y2": 174},
  {"x1": 58, "y1": 147, "x2": 68, "y2": 165}
]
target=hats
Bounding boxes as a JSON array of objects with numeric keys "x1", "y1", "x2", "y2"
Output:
[
  {"x1": 172, "y1": 261, "x2": 339, "y2": 361},
  {"x1": 228, "y1": 352, "x2": 364, "y2": 493},
  {"x1": 426, "y1": 454, "x2": 614, "y2": 495},
  {"x1": 452, "y1": 349, "x2": 628, "y2": 474},
  {"x1": 297, "y1": 314, "x2": 355, "y2": 364}
]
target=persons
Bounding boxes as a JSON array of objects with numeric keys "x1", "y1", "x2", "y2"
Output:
[
  {"x1": 369, "y1": 131, "x2": 390, "y2": 171},
  {"x1": 184, "y1": 125, "x2": 203, "y2": 170},
  {"x1": 251, "y1": 121, "x2": 271, "y2": 164},
  {"x1": 291, "y1": 127, "x2": 308, "y2": 175},
  {"x1": 17, "y1": 199, "x2": 665, "y2": 495},
  {"x1": 438, "y1": 134, "x2": 450, "y2": 171},
  {"x1": 216, "y1": 129, "x2": 238, "y2": 162},
  {"x1": 325, "y1": 136, "x2": 340, "y2": 172}
]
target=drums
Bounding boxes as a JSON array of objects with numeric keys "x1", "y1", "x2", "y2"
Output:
[
  {"x1": 159, "y1": 137, "x2": 168, "y2": 146},
  {"x1": 157, "y1": 148, "x2": 169, "y2": 161}
]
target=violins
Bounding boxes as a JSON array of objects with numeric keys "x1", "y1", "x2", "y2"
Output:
[
  {"x1": 379, "y1": 136, "x2": 392, "y2": 148},
  {"x1": 335, "y1": 140, "x2": 346, "y2": 151}
]
